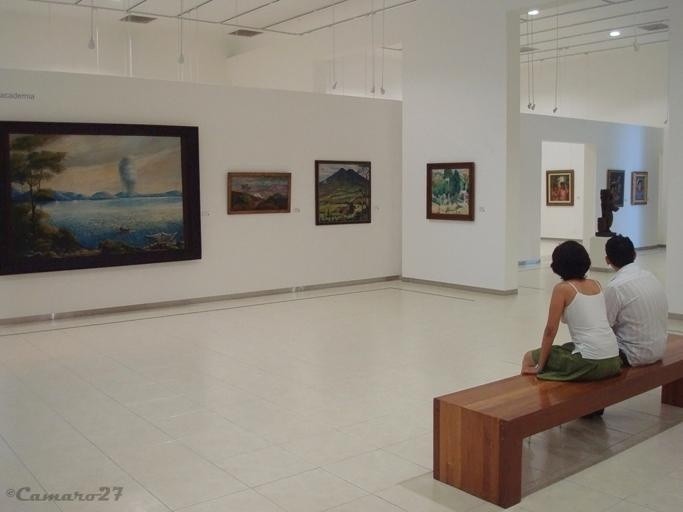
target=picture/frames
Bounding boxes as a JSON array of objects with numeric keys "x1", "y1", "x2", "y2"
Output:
[
  {"x1": 545, "y1": 169, "x2": 573, "y2": 206},
  {"x1": 314, "y1": 159, "x2": 371, "y2": 224},
  {"x1": 0, "y1": 120, "x2": 202, "y2": 276},
  {"x1": 425, "y1": 161, "x2": 475, "y2": 221},
  {"x1": 227, "y1": 171, "x2": 291, "y2": 215},
  {"x1": 605, "y1": 170, "x2": 648, "y2": 208}
]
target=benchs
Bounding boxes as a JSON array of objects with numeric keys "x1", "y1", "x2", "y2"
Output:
[{"x1": 432, "y1": 331, "x2": 682, "y2": 508}]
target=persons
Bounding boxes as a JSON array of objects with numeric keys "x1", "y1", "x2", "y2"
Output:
[
  {"x1": 522, "y1": 236, "x2": 668, "y2": 419},
  {"x1": 636, "y1": 180, "x2": 643, "y2": 200}
]
